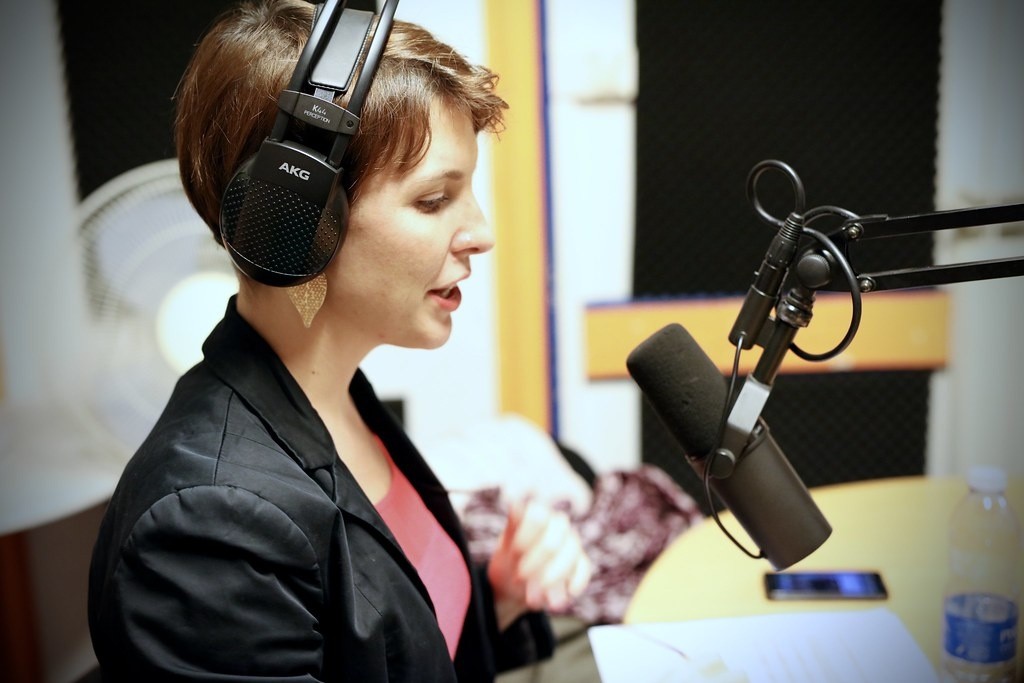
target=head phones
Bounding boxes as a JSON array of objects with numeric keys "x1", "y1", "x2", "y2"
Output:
[{"x1": 218, "y1": 0, "x2": 399, "y2": 285}]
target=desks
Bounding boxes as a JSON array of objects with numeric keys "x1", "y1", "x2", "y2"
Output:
[{"x1": 587, "y1": 473, "x2": 1024, "y2": 683}]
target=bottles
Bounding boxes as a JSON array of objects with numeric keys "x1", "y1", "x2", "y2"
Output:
[{"x1": 936, "y1": 467, "x2": 1018, "y2": 683}]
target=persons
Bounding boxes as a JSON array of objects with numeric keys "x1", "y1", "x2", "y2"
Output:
[{"x1": 88, "y1": 1, "x2": 593, "y2": 683}]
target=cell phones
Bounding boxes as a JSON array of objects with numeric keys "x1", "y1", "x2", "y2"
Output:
[{"x1": 764, "y1": 571, "x2": 888, "y2": 601}]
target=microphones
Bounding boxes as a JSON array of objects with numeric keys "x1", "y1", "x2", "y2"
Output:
[{"x1": 624, "y1": 324, "x2": 830, "y2": 572}]
